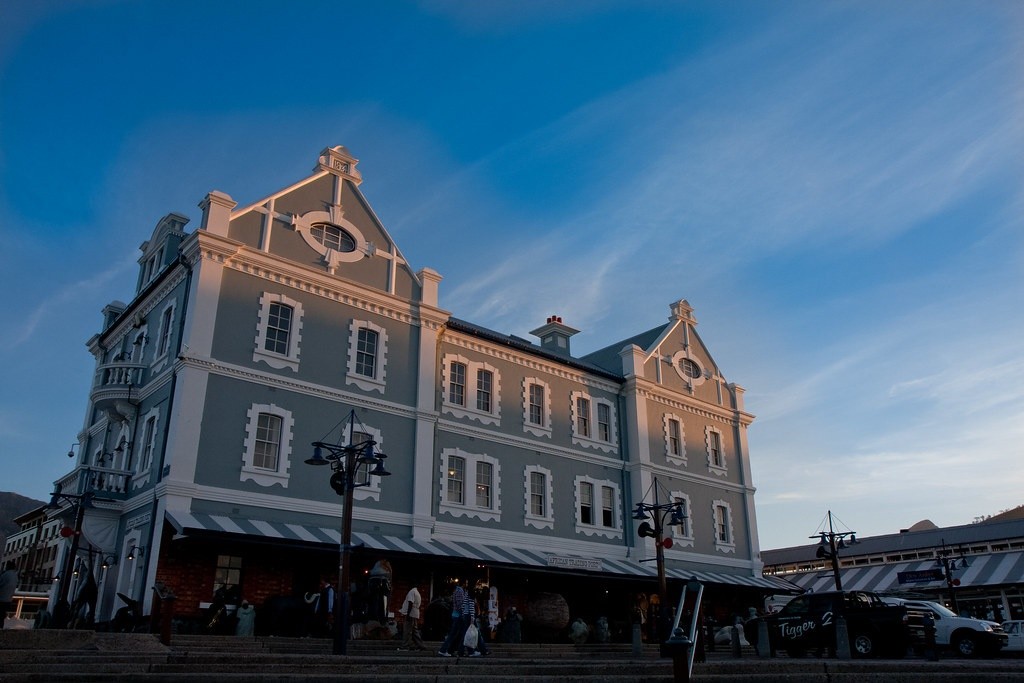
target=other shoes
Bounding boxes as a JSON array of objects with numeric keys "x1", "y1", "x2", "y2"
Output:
[
  {"x1": 482, "y1": 651, "x2": 491, "y2": 658},
  {"x1": 397, "y1": 647, "x2": 409, "y2": 652},
  {"x1": 456, "y1": 653, "x2": 466, "y2": 657},
  {"x1": 415, "y1": 647, "x2": 422, "y2": 652},
  {"x1": 437, "y1": 650, "x2": 452, "y2": 657},
  {"x1": 469, "y1": 651, "x2": 481, "y2": 657}
]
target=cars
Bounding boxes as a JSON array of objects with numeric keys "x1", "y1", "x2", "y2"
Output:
[
  {"x1": 998, "y1": 619, "x2": 1024, "y2": 656},
  {"x1": 873, "y1": 598, "x2": 1011, "y2": 657},
  {"x1": 743, "y1": 588, "x2": 918, "y2": 659}
]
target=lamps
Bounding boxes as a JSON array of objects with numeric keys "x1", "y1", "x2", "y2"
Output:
[
  {"x1": 115, "y1": 441, "x2": 133, "y2": 452},
  {"x1": 72, "y1": 564, "x2": 85, "y2": 574},
  {"x1": 51, "y1": 571, "x2": 65, "y2": 581},
  {"x1": 134, "y1": 311, "x2": 146, "y2": 327},
  {"x1": 117, "y1": 350, "x2": 132, "y2": 360},
  {"x1": 173, "y1": 532, "x2": 188, "y2": 540},
  {"x1": 125, "y1": 545, "x2": 145, "y2": 560},
  {"x1": 102, "y1": 555, "x2": 119, "y2": 569},
  {"x1": 133, "y1": 335, "x2": 149, "y2": 345},
  {"x1": 96, "y1": 453, "x2": 113, "y2": 462}
]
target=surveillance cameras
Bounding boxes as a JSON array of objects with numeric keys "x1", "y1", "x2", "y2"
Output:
[{"x1": 68, "y1": 451, "x2": 75, "y2": 457}]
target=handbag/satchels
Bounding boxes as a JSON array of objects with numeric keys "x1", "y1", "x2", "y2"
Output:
[
  {"x1": 434, "y1": 604, "x2": 452, "y2": 618},
  {"x1": 463, "y1": 624, "x2": 478, "y2": 649}
]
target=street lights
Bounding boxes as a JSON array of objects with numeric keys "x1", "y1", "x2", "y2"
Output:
[
  {"x1": 933, "y1": 540, "x2": 970, "y2": 617},
  {"x1": 629, "y1": 479, "x2": 690, "y2": 644},
  {"x1": 303, "y1": 409, "x2": 392, "y2": 656},
  {"x1": 44, "y1": 483, "x2": 118, "y2": 631},
  {"x1": 807, "y1": 510, "x2": 862, "y2": 660}
]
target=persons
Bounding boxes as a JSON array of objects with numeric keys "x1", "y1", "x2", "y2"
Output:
[
  {"x1": 572, "y1": 594, "x2": 659, "y2": 644},
  {"x1": 398, "y1": 579, "x2": 427, "y2": 652},
  {"x1": 209, "y1": 559, "x2": 393, "y2": 640},
  {"x1": 0, "y1": 560, "x2": 18, "y2": 629},
  {"x1": 438, "y1": 577, "x2": 523, "y2": 658}
]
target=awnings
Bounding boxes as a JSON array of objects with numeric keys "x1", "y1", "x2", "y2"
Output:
[
  {"x1": 162, "y1": 509, "x2": 805, "y2": 590},
  {"x1": 765, "y1": 549, "x2": 1024, "y2": 604}
]
